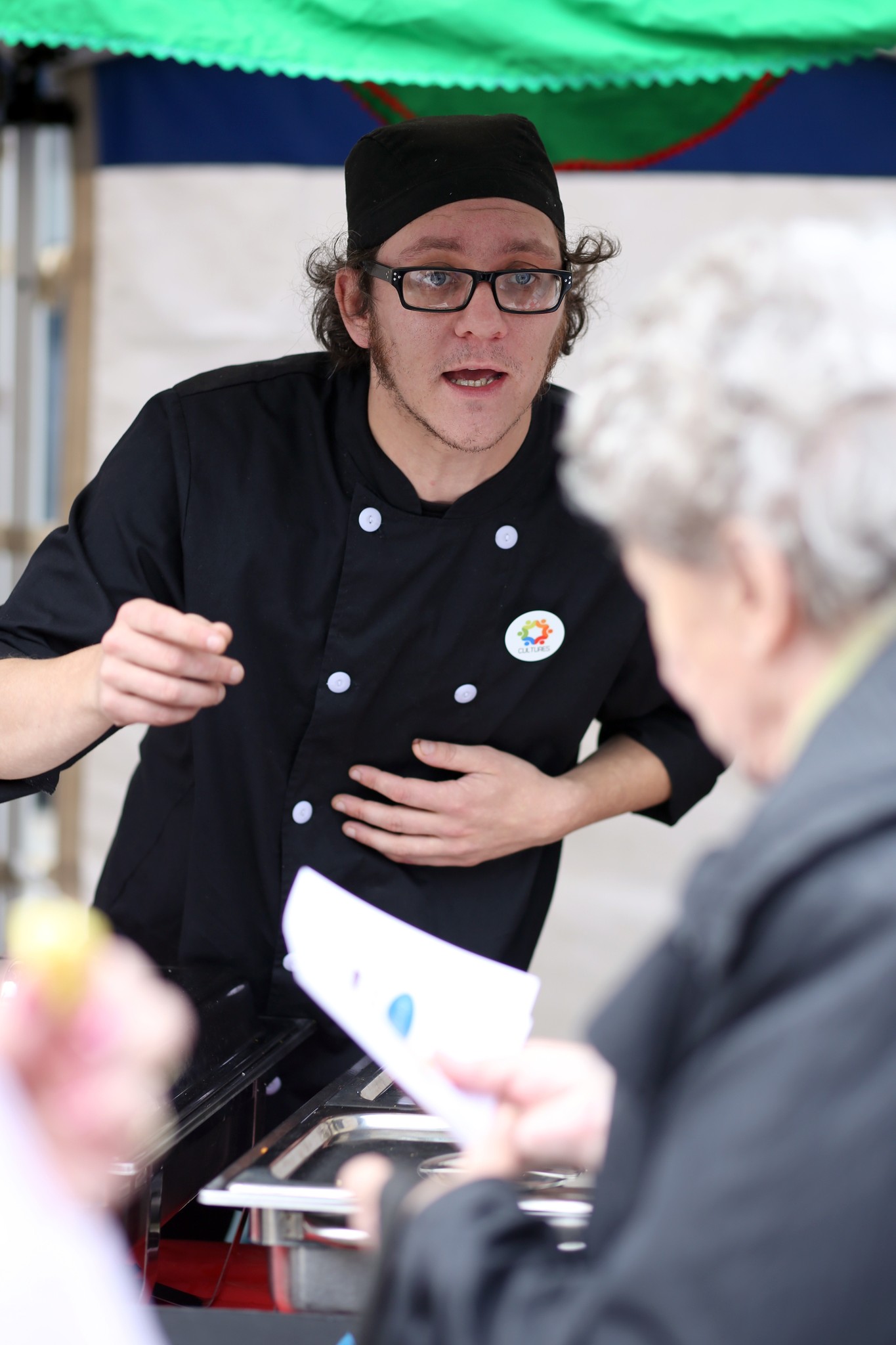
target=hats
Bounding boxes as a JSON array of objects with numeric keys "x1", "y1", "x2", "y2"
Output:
[{"x1": 344, "y1": 112, "x2": 565, "y2": 258}]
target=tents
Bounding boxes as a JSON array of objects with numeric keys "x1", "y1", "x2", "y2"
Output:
[{"x1": 0, "y1": 1, "x2": 896, "y2": 188}]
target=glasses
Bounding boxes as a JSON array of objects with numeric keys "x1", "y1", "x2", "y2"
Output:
[{"x1": 361, "y1": 259, "x2": 572, "y2": 314}]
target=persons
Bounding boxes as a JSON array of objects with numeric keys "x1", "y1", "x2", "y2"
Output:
[
  {"x1": 0, "y1": 109, "x2": 739, "y2": 1310},
  {"x1": 333, "y1": 193, "x2": 896, "y2": 1345},
  {"x1": 0, "y1": 847, "x2": 209, "y2": 1345}
]
